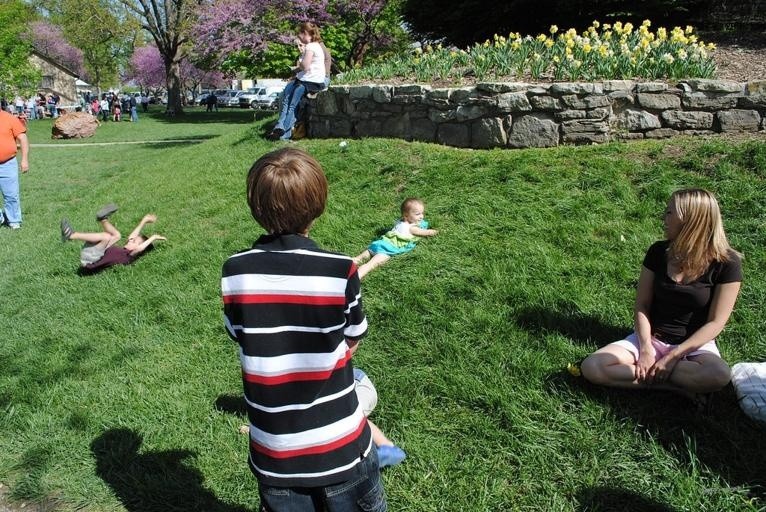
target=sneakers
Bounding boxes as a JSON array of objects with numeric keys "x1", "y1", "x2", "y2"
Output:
[
  {"x1": 8, "y1": 223, "x2": 20, "y2": 229},
  {"x1": 374, "y1": 445, "x2": 407, "y2": 468},
  {"x1": 267, "y1": 128, "x2": 285, "y2": 141}
]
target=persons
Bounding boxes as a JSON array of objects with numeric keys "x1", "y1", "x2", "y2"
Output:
[
  {"x1": 81, "y1": 91, "x2": 148, "y2": 122},
  {"x1": 581, "y1": 190, "x2": 742, "y2": 395},
  {"x1": 354, "y1": 369, "x2": 406, "y2": 467},
  {"x1": 206, "y1": 92, "x2": 218, "y2": 110},
  {"x1": 0, "y1": 93, "x2": 58, "y2": 228},
  {"x1": 355, "y1": 199, "x2": 437, "y2": 278},
  {"x1": 221, "y1": 148, "x2": 388, "y2": 511},
  {"x1": 267, "y1": 20, "x2": 332, "y2": 141},
  {"x1": 61, "y1": 206, "x2": 167, "y2": 274}
]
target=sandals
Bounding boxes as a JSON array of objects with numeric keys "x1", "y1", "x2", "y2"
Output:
[
  {"x1": 61, "y1": 218, "x2": 74, "y2": 243},
  {"x1": 96, "y1": 204, "x2": 117, "y2": 220}
]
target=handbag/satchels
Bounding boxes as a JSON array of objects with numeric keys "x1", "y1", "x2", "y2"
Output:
[{"x1": 731, "y1": 361, "x2": 765, "y2": 421}]
